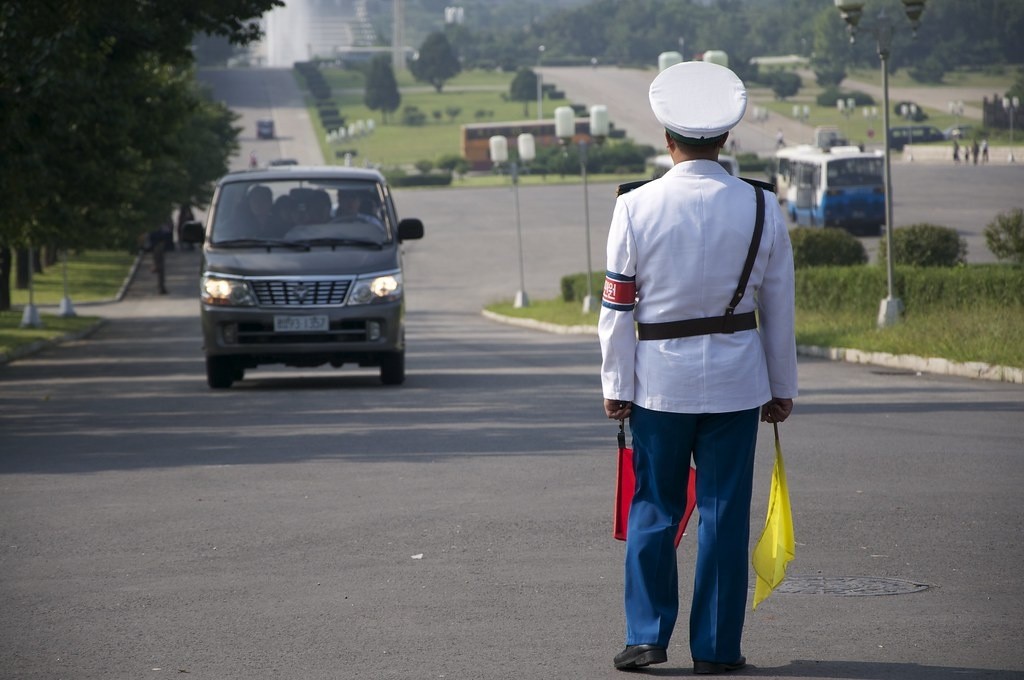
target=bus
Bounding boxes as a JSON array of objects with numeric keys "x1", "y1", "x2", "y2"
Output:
[{"x1": 775, "y1": 141, "x2": 887, "y2": 234}]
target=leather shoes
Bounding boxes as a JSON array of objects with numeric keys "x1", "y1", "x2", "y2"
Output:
[
  {"x1": 614, "y1": 643, "x2": 669, "y2": 670},
  {"x1": 691, "y1": 656, "x2": 747, "y2": 674}
]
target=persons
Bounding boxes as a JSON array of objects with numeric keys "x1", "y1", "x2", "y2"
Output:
[
  {"x1": 769, "y1": 122, "x2": 789, "y2": 147},
  {"x1": 594, "y1": 60, "x2": 801, "y2": 673},
  {"x1": 945, "y1": 136, "x2": 990, "y2": 165},
  {"x1": 221, "y1": 186, "x2": 382, "y2": 242}
]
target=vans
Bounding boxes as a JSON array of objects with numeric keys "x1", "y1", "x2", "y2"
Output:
[
  {"x1": 198, "y1": 165, "x2": 425, "y2": 389},
  {"x1": 888, "y1": 123, "x2": 945, "y2": 151}
]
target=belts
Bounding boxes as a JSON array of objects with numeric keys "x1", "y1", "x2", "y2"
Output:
[{"x1": 636, "y1": 310, "x2": 759, "y2": 342}]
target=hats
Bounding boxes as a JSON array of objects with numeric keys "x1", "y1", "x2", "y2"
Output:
[{"x1": 649, "y1": 60, "x2": 748, "y2": 142}]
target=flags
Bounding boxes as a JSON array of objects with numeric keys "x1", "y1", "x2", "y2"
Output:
[
  {"x1": 751, "y1": 445, "x2": 800, "y2": 609},
  {"x1": 615, "y1": 447, "x2": 696, "y2": 548}
]
